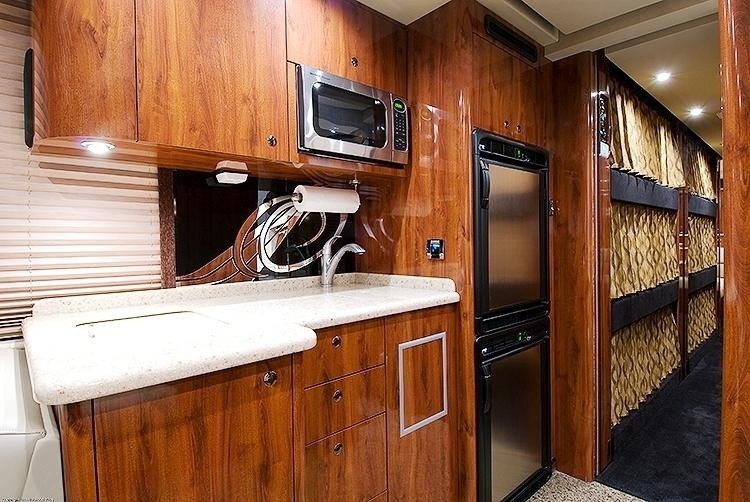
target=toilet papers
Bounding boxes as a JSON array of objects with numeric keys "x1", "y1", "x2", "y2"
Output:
[{"x1": 293, "y1": 185, "x2": 360, "y2": 214}]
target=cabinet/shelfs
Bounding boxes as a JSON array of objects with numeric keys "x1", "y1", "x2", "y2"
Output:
[
  {"x1": 92, "y1": 354, "x2": 294, "y2": 502},
  {"x1": 293, "y1": 316, "x2": 389, "y2": 502},
  {"x1": 385, "y1": 302, "x2": 465, "y2": 501},
  {"x1": 26, "y1": 1, "x2": 289, "y2": 180},
  {"x1": 406, "y1": 1, "x2": 547, "y2": 145},
  {"x1": 285, "y1": 0, "x2": 408, "y2": 97}
]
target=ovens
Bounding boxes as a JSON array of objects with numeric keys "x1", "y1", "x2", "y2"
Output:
[{"x1": 472, "y1": 130, "x2": 553, "y2": 500}]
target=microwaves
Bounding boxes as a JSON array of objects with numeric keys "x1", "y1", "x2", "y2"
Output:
[{"x1": 296, "y1": 66, "x2": 409, "y2": 171}]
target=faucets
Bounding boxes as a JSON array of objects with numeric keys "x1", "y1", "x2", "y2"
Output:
[{"x1": 322, "y1": 235, "x2": 367, "y2": 286}]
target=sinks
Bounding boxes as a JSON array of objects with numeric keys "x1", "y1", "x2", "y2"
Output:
[{"x1": 74, "y1": 309, "x2": 239, "y2": 357}]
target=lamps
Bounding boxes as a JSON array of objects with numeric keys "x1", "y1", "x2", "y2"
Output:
[{"x1": 214, "y1": 169, "x2": 251, "y2": 185}]
